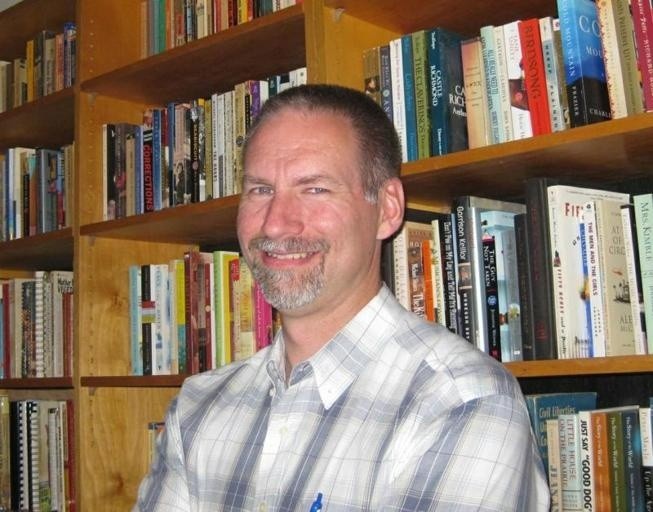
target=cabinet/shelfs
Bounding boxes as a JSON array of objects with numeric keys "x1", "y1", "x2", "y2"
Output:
[{"x1": 0, "y1": 1, "x2": 650, "y2": 507}]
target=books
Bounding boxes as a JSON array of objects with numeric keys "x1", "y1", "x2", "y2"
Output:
[
  {"x1": 140, "y1": 1, "x2": 297, "y2": 58},
  {"x1": 526, "y1": 391, "x2": 652, "y2": 512},
  {"x1": 100, "y1": 67, "x2": 307, "y2": 221},
  {"x1": 0, "y1": 394, "x2": 76, "y2": 512},
  {"x1": 380, "y1": 175, "x2": 653, "y2": 363},
  {"x1": 2, "y1": 270, "x2": 75, "y2": 378},
  {"x1": 1, "y1": 22, "x2": 78, "y2": 110},
  {"x1": 360, "y1": 2, "x2": 652, "y2": 162},
  {"x1": 129, "y1": 249, "x2": 282, "y2": 375},
  {"x1": 1, "y1": 141, "x2": 75, "y2": 241}
]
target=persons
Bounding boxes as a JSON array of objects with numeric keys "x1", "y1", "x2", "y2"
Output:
[{"x1": 123, "y1": 84, "x2": 558, "y2": 512}]
